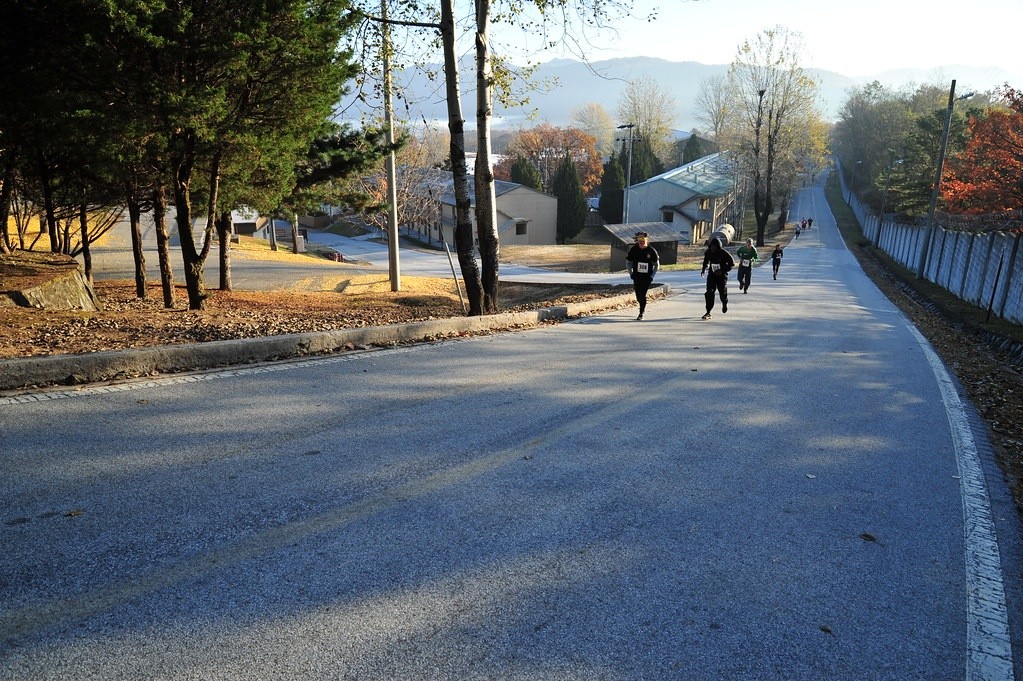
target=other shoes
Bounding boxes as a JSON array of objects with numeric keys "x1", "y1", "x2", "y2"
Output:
[
  {"x1": 739, "y1": 283, "x2": 743, "y2": 290},
  {"x1": 637, "y1": 314, "x2": 643, "y2": 320},
  {"x1": 722, "y1": 305, "x2": 727, "y2": 313},
  {"x1": 744, "y1": 289, "x2": 747, "y2": 294},
  {"x1": 702, "y1": 313, "x2": 712, "y2": 319}
]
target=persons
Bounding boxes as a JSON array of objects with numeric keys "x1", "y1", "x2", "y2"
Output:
[
  {"x1": 771, "y1": 244, "x2": 783, "y2": 280},
  {"x1": 625, "y1": 232, "x2": 660, "y2": 322},
  {"x1": 700, "y1": 237, "x2": 735, "y2": 320},
  {"x1": 808, "y1": 216, "x2": 813, "y2": 228},
  {"x1": 736, "y1": 237, "x2": 759, "y2": 294},
  {"x1": 795, "y1": 224, "x2": 801, "y2": 240},
  {"x1": 812, "y1": 172, "x2": 815, "y2": 184},
  {"x1": 801, "y1": 217, "x2": 807, "y2": 231}
]
